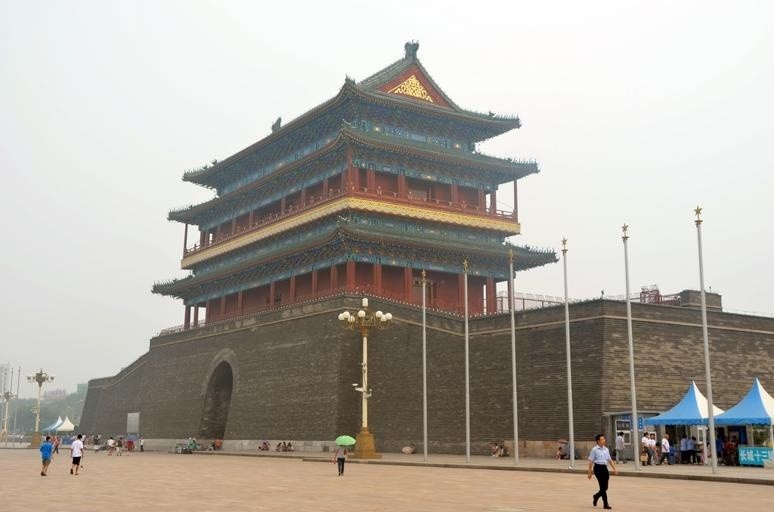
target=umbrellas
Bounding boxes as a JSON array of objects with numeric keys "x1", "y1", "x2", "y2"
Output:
[{"x1": 335, "y1": 435, "x2": 357, "y2": 446}]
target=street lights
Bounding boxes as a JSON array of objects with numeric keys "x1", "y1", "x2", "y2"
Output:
[
  {"x1": 337, "y1": 297, "x2": 393, "y2": 459},
  {"x1": 27, "y1": 368, "x2": 55, "y2": 448}
]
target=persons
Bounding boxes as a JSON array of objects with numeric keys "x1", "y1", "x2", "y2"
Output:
[
  {"x1": 107, "y1": 437, "x2": 145, "y2": 456},
  {"x1": 81, "y1": 433, "x2": 102, "y2": 444},
  {"x1": 333, "y1": 445, "x2": 347, "y2": 475},
  {"x1": 258, "y1": 441, "x2": 294, "y2": 451},
  {"x1": 187, "y1": 437, "x2": 214, "y2": 451},
  {"x1": 51, "y1": 436, "x2": 59, "y2": 454},
  {"x1": 615, "y1": 431, "x2": 739, "y2": 466},
  {"x1": 39, "y1": 436, "x2": 53, "y2": 476},
  {"x1": 70, "y1": 435, "x2": 84, "y2": 475},
  {"x1": 492, "y1": 443, "x2": 505, "y2": 457},
  {"x1": 556, "y1": 447, "x2": 562, "y2": 461},
  {"x1": 588, "y1": 435, "x2": 618, "y2": 509}
]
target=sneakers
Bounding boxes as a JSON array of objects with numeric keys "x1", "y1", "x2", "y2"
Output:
[{"x1": 593, "y1": 495, "x2": 612, "y2": 509}]
